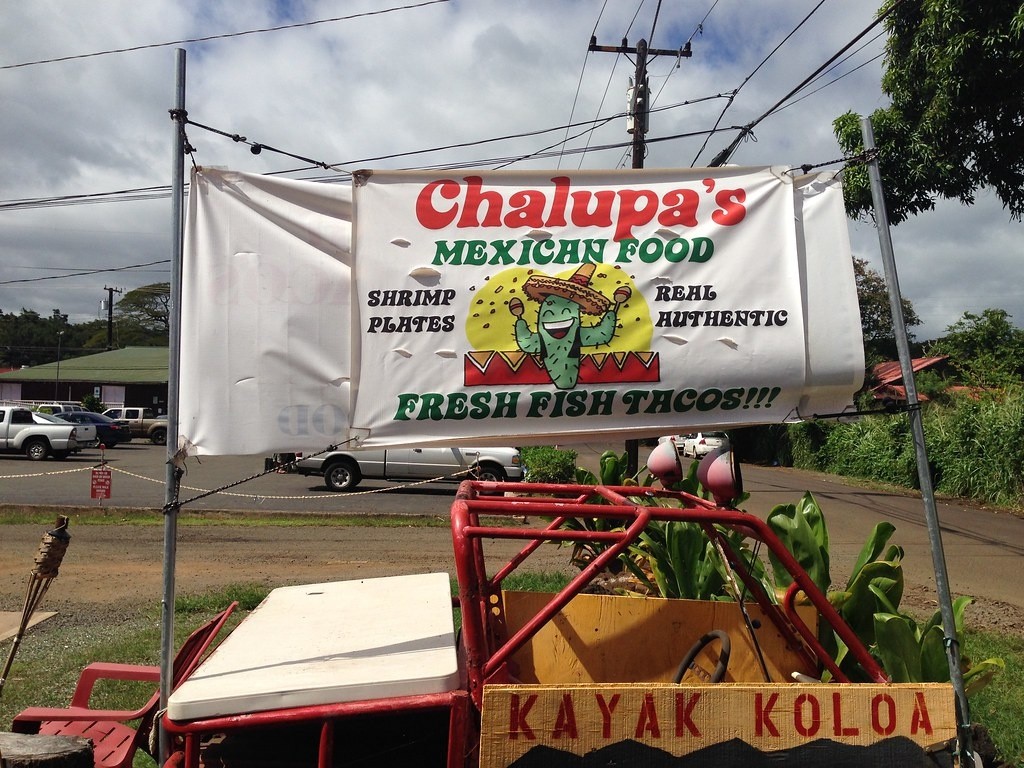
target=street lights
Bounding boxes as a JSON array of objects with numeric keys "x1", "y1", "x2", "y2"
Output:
[{"x1": 54, "y1": 330, "x2": 65, "y2": 400}]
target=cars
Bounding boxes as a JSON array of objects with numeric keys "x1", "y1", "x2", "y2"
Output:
[
  {"x1": 53, "y1": 411, "x2": 132, "y2": 448},
  {"x1": 683, "y1": 430, "x2": 730, "y2": 459},
  {"x1": 293, "y1": 445, "x2": 525, "y2": 495},
  {"x1": 657, "y1": 433, "x2": 693, "y2": 454},
  {"x1": 31, "y1": 412, "x2": 69, "y2": 424}
]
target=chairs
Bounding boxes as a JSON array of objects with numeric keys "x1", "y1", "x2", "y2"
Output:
[{"x1": 14, "y1": 599, "x2": 240, "y2": 768}]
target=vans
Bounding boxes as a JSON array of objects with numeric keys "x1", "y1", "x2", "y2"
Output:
[{"x1": 35, "y1": 404, "x2": 92, "y2": 414}]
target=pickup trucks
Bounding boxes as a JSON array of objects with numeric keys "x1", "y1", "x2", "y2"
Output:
[{"x1": 0, "y1": 406, "x2": 97, "y2": 460}]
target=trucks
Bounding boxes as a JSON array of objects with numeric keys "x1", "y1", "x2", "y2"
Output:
[{"x1": 101, "y1": 407, "x2": 168, "y2": 445}]
